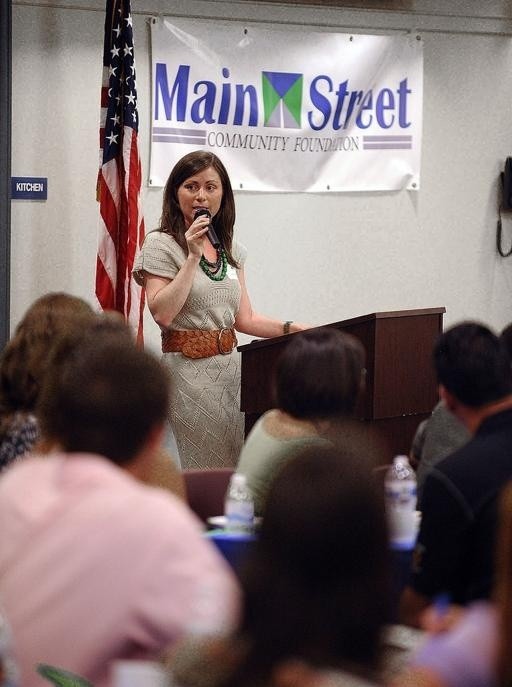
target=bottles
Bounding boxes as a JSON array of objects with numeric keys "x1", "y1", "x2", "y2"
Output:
[
  {"x1": 383, "y1": 453, "x2": 421, "y2": 551},
  {"x1": 223, "y1": 468, "x2": 258, "y2": 543}
]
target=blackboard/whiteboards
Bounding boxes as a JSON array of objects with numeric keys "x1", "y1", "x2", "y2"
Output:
[{"x1": 9, "y1": 0, "x2": 512, "y2": 365}]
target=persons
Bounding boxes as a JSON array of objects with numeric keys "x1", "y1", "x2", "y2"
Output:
[
  {"x1": 1, "y1": 288, "x2": 198, "y2": 496},
  {"x1": 397, "y1": 319, "x2": 511, "y2": 627},
  {"x1": 2, "y1": 334, "x2": 242, "y2": 687},
  {"x1": 163, "y1": 434, "x2": 432, "y2": 687},
  {"x1": 377, "y1": 477, "x2": 512, "y2": 687},
  {"x1": 133, "y1": 150, "x2": 312, "y2": 470},
  {"x1": 406, "y1": 320, "x2": 511, "y2": 513},
  {"x1": 220, "y1": 329, "x2": 372, "y2": 525}
]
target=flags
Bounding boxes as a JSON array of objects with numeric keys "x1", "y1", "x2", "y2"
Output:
[{"x1": 95, "y1": 0, "x2": 148, "y2": 343}]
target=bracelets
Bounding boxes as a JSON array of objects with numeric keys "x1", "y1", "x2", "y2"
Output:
[{"x1": 282, "y1": 319, "x2": 295, "y2": 334}]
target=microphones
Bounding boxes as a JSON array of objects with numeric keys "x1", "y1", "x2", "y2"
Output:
[{"x1": 192, "y1": 209, "x2": 219, "y2": 249}]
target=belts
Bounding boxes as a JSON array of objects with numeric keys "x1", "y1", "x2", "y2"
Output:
[{"x1": 160, "y1": 327, "x2": 238, "y2": 360}]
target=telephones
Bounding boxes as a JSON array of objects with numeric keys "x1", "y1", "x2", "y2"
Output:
[{"x1": 498, "y1": 156, "x2": 512, "y2": 215}]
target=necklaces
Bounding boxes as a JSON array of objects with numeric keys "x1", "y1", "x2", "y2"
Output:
[{"x1": 195, "y1": 244, "x2": 229, "y2": 282}]
